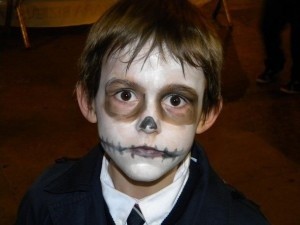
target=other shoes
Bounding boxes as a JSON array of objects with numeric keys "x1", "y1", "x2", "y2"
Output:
[
  {"x1": 280, "y1": 83, "x2": 300, "y2": 93},
  {"x1": 256, "y1": 69, "x2": 277, "y2": 84}
]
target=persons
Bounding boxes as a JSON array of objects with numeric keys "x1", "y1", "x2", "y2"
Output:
[
  {"x1": 256, "y1": 0, "x2": 300, "y2": 94},
  {"x1": 16, "y1": 0, "x2": 272, "y2": 225}
]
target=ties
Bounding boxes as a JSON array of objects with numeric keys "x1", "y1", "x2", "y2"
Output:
[{"x1": 126, "y1": 204, "x2": 146, "y2": 225}]
